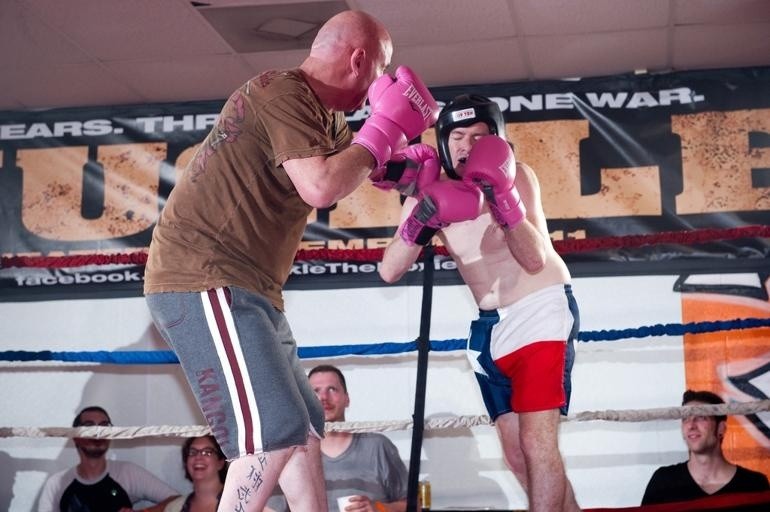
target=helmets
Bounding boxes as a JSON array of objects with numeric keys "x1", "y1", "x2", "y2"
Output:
[{"x1": 436, "y1": 93, "x2": 506, "y2": 178}]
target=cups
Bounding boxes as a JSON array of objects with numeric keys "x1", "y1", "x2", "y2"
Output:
[{"x1": 336, "y1": 495, "x2": 358, "y2": 512}]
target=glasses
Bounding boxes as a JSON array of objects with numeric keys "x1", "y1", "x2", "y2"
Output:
[{"x1": 187, "y1": 447, "x2": 219, "y2": 456}]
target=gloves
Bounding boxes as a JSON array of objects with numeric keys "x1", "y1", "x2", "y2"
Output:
[
  {"x1": 368, "y1": 134, "x2": 526, "y2": 245},
  {"x1": 351, "y1": 66, "x2": 439, "y2": 169}
]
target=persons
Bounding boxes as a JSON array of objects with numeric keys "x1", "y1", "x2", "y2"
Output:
[
  {"x1": 143, "y1": 10, "x2": 442, "y2": 512},
  {"x1": 640, "y1": 389, "x2": 770, "y2": 512},
  {"x1": 117, "y1": 436, "x2": 279, "y2": 512},
  {"x1": 38, "y1": 406, "x2": 185, "y2": 512},
  {"x1": 286, "y1": 365, "x2": 422, "y2": 512},
  {"x1": 378, "y1": 94, "x2": 581, "y2": 512}
]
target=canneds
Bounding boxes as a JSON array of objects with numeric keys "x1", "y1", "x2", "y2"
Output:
[{"x1": 418, "y1": 482, "x2": 431, "y2": 510}]
